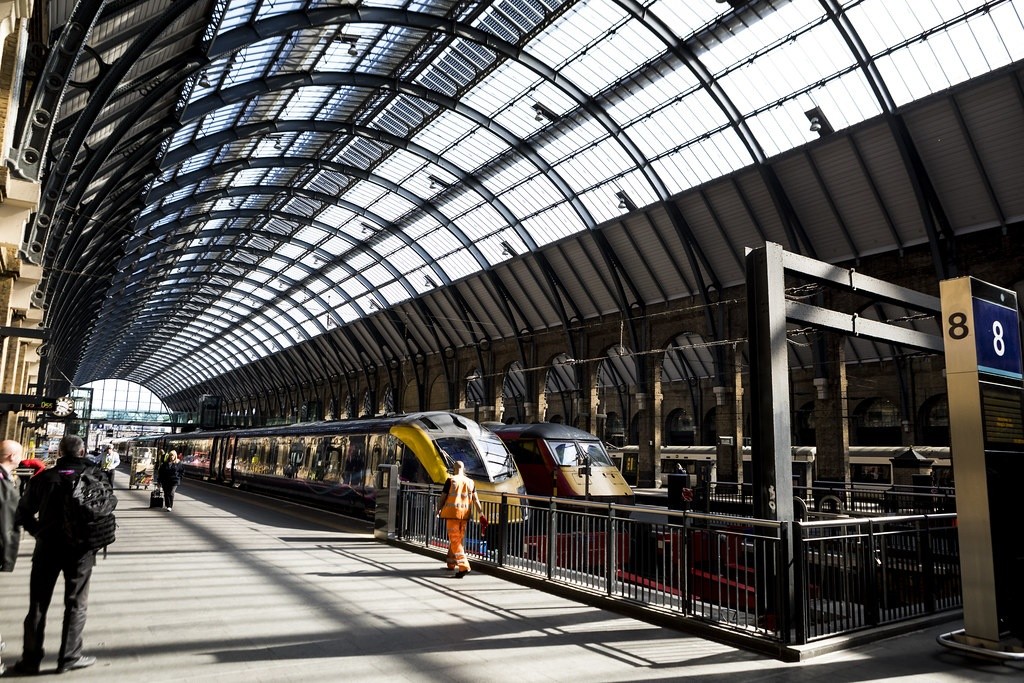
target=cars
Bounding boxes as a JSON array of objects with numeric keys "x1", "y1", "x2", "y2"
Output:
[{"x1": 40, "y1": 446, "x2": 48, "y2": 450}]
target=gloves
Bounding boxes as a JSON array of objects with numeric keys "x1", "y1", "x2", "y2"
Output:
[
  {"x1": 481, "y1": 523, "x2": 487, "y2": 537},
  {"x1": 479, "y1": 511, "x2": 488, "y2": 525}
]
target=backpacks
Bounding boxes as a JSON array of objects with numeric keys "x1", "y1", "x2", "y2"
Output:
[{"x1": 39, "y1": 462, "x2": 120, "y2": 565}]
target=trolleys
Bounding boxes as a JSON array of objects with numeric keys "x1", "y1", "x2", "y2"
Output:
[{"x1": 129, "y1": 447, "x2": 158, "y2": 490}]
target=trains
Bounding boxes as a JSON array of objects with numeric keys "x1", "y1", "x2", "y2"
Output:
[
  {"x1": 90, "y1": 411, "x2": 529, "y2": 554},
  {"x1": 480, "y1": 420, "x2": 636, "y2": 529},
  {"x1": 606, "y1": 443, "x2": 956, "y2": 505}
]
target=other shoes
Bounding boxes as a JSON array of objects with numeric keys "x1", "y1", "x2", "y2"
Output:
[
  {"x1": 455, "y1": 568, "x2": 471, "y2": 578},
  {"x1": 56, "y1": 655, "x2": 97, "y2": 673},
  {"x1": 164, "y1": 506, "x2": 172, "y2": 511},
  {"x1": 7, "y1": 664, "x2": 19, "y2": 675}
]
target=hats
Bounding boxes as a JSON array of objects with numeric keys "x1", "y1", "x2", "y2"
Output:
[{"x1": 106, "y1": 444, "x2": 115, "y2": 450}]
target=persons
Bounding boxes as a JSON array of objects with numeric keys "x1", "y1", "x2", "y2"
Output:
[
  {"x1": 158, "y1": 449, "x2": 169, "y2": 470},
  {"x1": 434, "y1": 460, "x2": 484, "y2": 578},
  {"x1": 90, "y1": 447, "x2": 102, "y2": 457},
  {"x1": 156, "y1": 450, "x2": 184, "y2": 511},
  {"x1": 101, "y1": 446, "x2": 120, "y2": 488},
  {"x1": 14, "y1": 435, "x2": 113, "y2": 672},
  {"x1": 0, "y1": 439, "x2": 22, "y2": 678}
]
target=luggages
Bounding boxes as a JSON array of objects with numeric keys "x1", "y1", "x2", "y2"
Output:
[{"x1": 149, "y1": 483, "x2": 164, "y2": 508}]
